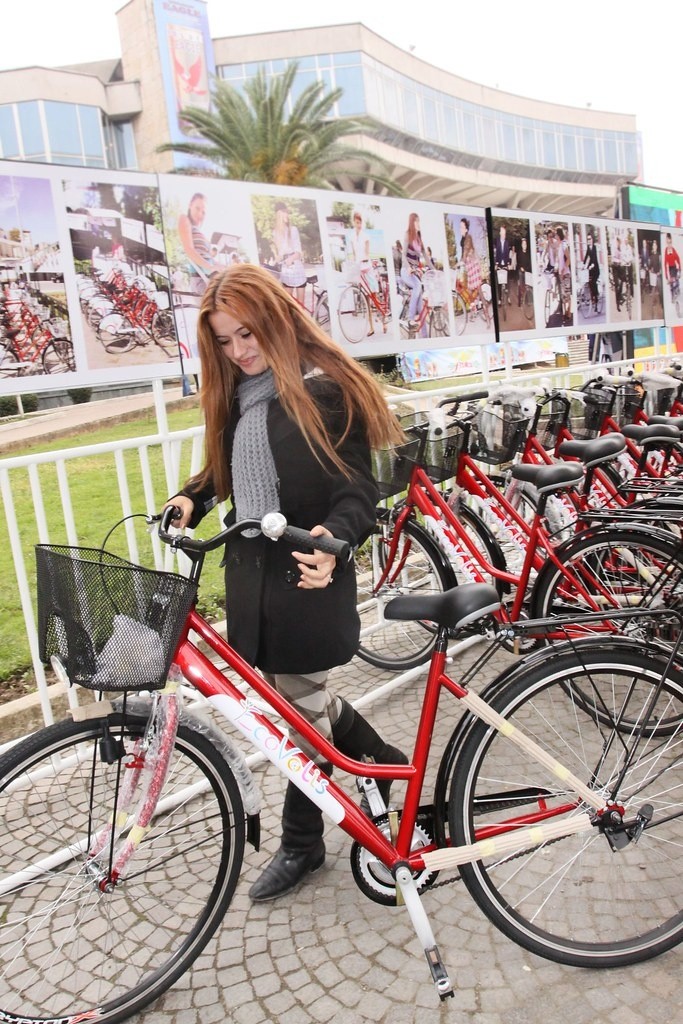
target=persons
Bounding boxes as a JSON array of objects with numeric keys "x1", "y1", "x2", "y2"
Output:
[
  {"x1": 350, "y1": 212, "x2": 388, "y2": 336},
  {"x1": 393, "y1": 213, "x2": 435, "y2": 338},
  {"x1": 178, "y1": 192, "x2": 243, "y2": 297},
  {"x1": 271, "y1": 201, "x2": 307, "y2": 308},
  {"x1": 162, "y1": 265, "x2": 411, "y2": 900},
  {"x1": 589, "y1": 331, "x2": 624, "y2": 378},
  {"x1": 537, "y1": 225, "x2": 681, "y2": 320},
  {"x1": 454, "y1": 217, "x2": 531, "y2": 327},
  {"x1": 181, "y1": 375, "x2": 195, "y2": 396},
  {"x1": 112, "y1": 236, "x2": 128, "y2": 262}
]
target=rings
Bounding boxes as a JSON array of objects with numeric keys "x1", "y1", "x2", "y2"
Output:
[{"x1": 327, "y1": 575, "x2": 333, "y2": 583}]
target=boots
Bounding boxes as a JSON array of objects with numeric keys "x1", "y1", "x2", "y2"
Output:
[
  {"x1": 331, "y1": 694, "x2": 409, "y2": 820},
  {"x1": 247, "y1": 762, "x2": 333, "y2": 902}
]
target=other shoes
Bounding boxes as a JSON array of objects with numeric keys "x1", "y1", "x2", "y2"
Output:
[
  {"x1": 508, "y1": 298, "x2": 511, "y2": 306},
  {"x1": 498, "y1": 300, "x2": 501, "y2": 306},
  {"x1": 409, "y1": 321, "x2": 418, "y2": 328},
  {"x1": 367, "y1": 330, "x2": 374, "y2": 336}
]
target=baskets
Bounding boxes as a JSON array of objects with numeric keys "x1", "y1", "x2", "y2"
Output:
[
  {"x1": 649, "y1": 273, "x2": 658, "y2": 286},
  {"x1": 342, "y1": 260, "x2": 359, "y2": 283},
  {"x1": 12, "y1": 287, "x2": 69, "y2": 338},
  {"x1": 449, "y1": 268, "x2": 457, "y2": 290},
  {"x1": 541, "y1": 274, "x2": 552, "y2": 289},
  {"x1": 497, "y1": 268, "x2": 508, "y2": 285},
  {"x1": 641, "y1": 269, "x2": 645, "y2": 278},
  {"x1": 420, "y1": 269, "x2": 446, "y2": 307},
  {"x1": 36, "y1": 544, "x2": 201, "y2": 690},
  {"x1": 262, "y1": 267, "x2": 280, "y2": 282},
  {"x1": 524, "y1": 271, "x2": 533, "y2": 287},
  {"x1": 117, "y1": 262, "x2": 169, "y2": 310},
  {"x1": 367, "y1": 367, "x2": 681, "y2": 500},
  {"x1": 578, "y1": 269, "x2": 590, "y2": 282}
]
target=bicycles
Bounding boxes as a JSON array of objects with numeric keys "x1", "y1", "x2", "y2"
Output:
[
  {"x1": 0, "y1": 281, "x2": 76, "y2": 377},
  {"x1": 76, "y1": 261, "x2": 178, "y2": 354},
  {"x1": 494, "y1": 260, "x2": 511, "y2": 319},
  {"x1": 665, "y1": 272, "x2": 681, "y2": 318},
  {"x1": 540, "y1": 266, "x2": 572, "y2": 324},
  {"x1": 338, "y1": 259, "x2": 392, "y2": 343},
  {"x1": 0, "y1": 506, "x2": 683, "y2": 1024},
  {"x1": 349, "y1": 358, "x2": 683, "y2": 738},
  {"x1": 517, "y1": 267, "x2": 534, "y2": 321},
  {"x1": 609, "y1": 261, "x2": 634, "y2": 318},
  {"x1": 285, "y1": 273, "x2": 331, "y2": 335},
  {"x1": 395, "y1": 265, "x2": 494, "y2": 342},
  {"x1": 576, "y1": 262, "x2": 605, "y2": 320}
]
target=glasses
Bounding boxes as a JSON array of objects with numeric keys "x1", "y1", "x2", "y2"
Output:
[{"x1": 587, "y1": 238, "x2": 590, "y2": 240}]
